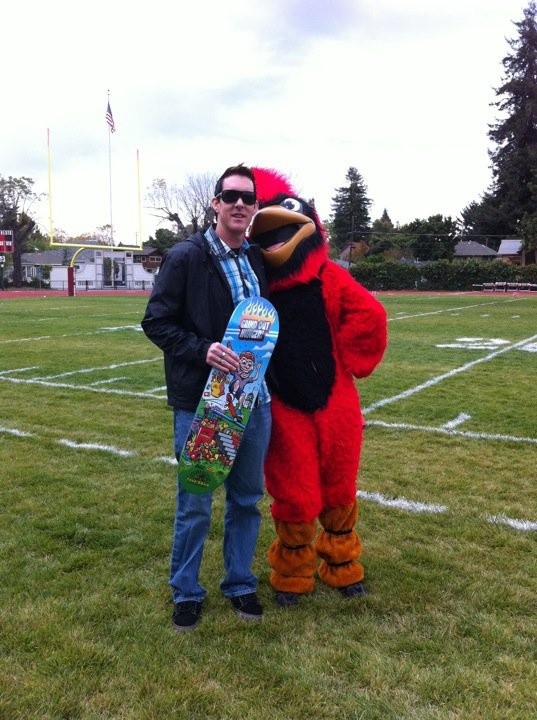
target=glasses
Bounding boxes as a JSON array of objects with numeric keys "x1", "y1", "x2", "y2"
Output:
[{"x1": 215, "y1": 190, "x2": 257, "y2": 205}]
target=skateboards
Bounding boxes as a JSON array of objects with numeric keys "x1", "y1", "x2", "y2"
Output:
[{"x1": 178, "y1": 295, "x2": 282, "y2": 496}]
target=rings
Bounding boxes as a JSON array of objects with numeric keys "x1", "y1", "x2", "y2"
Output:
[
  {"x1": 218, "y1": 360, "x2": 224, "y2": 365},
  {"x1": 220, "y1": 352, "x2": 225, "y2": 358}
]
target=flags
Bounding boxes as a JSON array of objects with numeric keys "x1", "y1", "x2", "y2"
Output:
[{"x1": 105, "y1": 100, "x2": 117, "y2": 136}]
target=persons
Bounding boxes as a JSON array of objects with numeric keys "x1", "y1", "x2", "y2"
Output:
[{"x1": 141, "y1": 164, "x2": 282, "y2": 628}]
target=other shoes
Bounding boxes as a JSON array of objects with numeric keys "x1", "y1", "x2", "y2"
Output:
[
  {"x1": 172, "y1": 601, "x2": 204, "y2": 630},
  {"x1": 228, "y1": 594, "x2": 265, "y2": 621}
]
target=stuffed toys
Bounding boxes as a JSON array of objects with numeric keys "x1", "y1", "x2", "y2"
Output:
[{"x1": 246, "y1": 167, "x2": 388, "y2": 608}]
